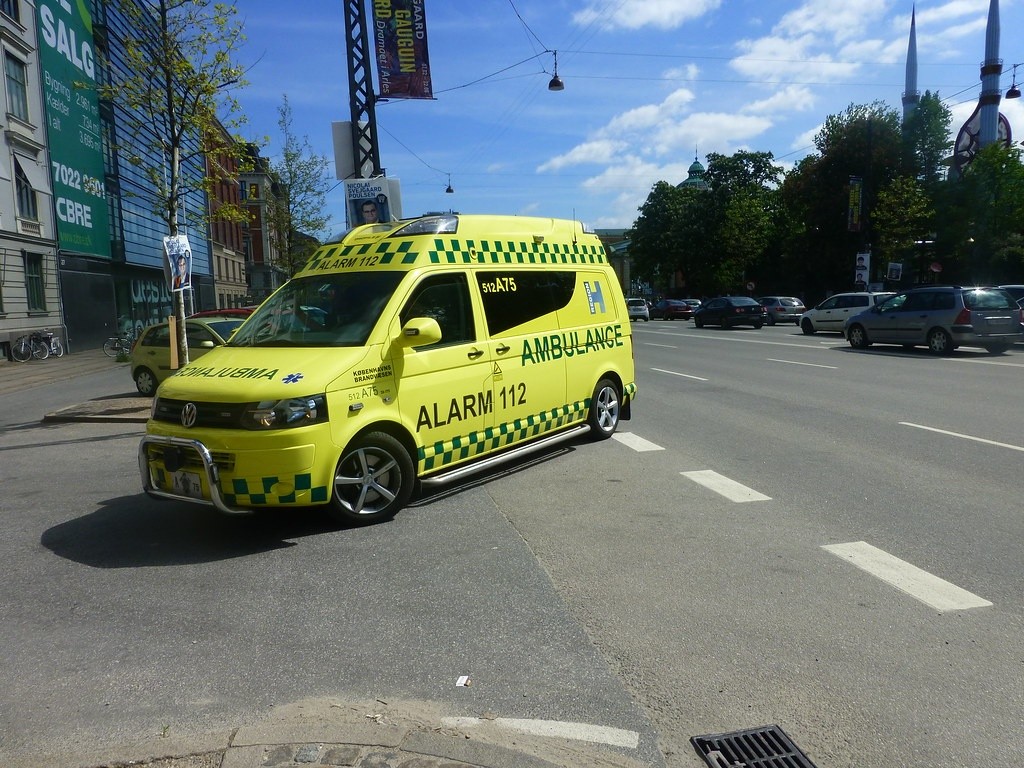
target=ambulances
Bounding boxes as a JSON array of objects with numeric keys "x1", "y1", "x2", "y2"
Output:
[{"x1": 137, "y1": 215, "x2": 636, "y2": 521}]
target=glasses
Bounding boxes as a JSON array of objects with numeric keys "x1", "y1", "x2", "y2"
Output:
[
  {"x1": 363, "y1": 209, "x2": 377, "y2": 213},
  {"x1": 179, "y1": 263, "x2": 186, "y2": 266}
]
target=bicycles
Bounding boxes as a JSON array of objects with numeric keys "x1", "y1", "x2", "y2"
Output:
[
  {"x1": 104, "y1": 327, "x2": 141, "y2": 357},
  {"x1": 11, "y1": 331, "x2": 64, "y2": 360}
]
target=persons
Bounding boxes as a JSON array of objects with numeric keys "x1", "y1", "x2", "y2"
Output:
[
  {"x1": 174, "y1": 256, "x2": 189, "y2": 289},
  {"x1": 856, "y1": 257, "x2": 867, "y2": 270},
  {"x1": 856, "y1": 273, "x2": 867, "y2": 288},
  {"x1": 354, "y1": 200, "x2": 386, "y2": 226}
]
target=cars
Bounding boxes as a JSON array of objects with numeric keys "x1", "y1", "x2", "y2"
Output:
[
  {"x1": 625, "y1": 298, "x2": 649, "y2": 322},
  {"x1": 799, "y1": 288, "x2": 905, "y2": 335},
  {"x1": 844, "y1": 286, "x2": 1024, "y2": 356},
  {"x1": 692, "y1": 296, "x2": 769, "y2": 330},
  {"x1": 130, "y1": 317, "x2": 274, "y2": 395},
  {"x1": 683, "y1": 299, "x2": 702, "y2": 312},
  {"x1": 181, "y1": 307, "x2": 317, "y2": 332},
  {"x1": 650, "y1": 300, "x2": 692, "y2": 322},
  {"x1": 756, "y1": 297, "x2": 808, "y2": 325}
]
